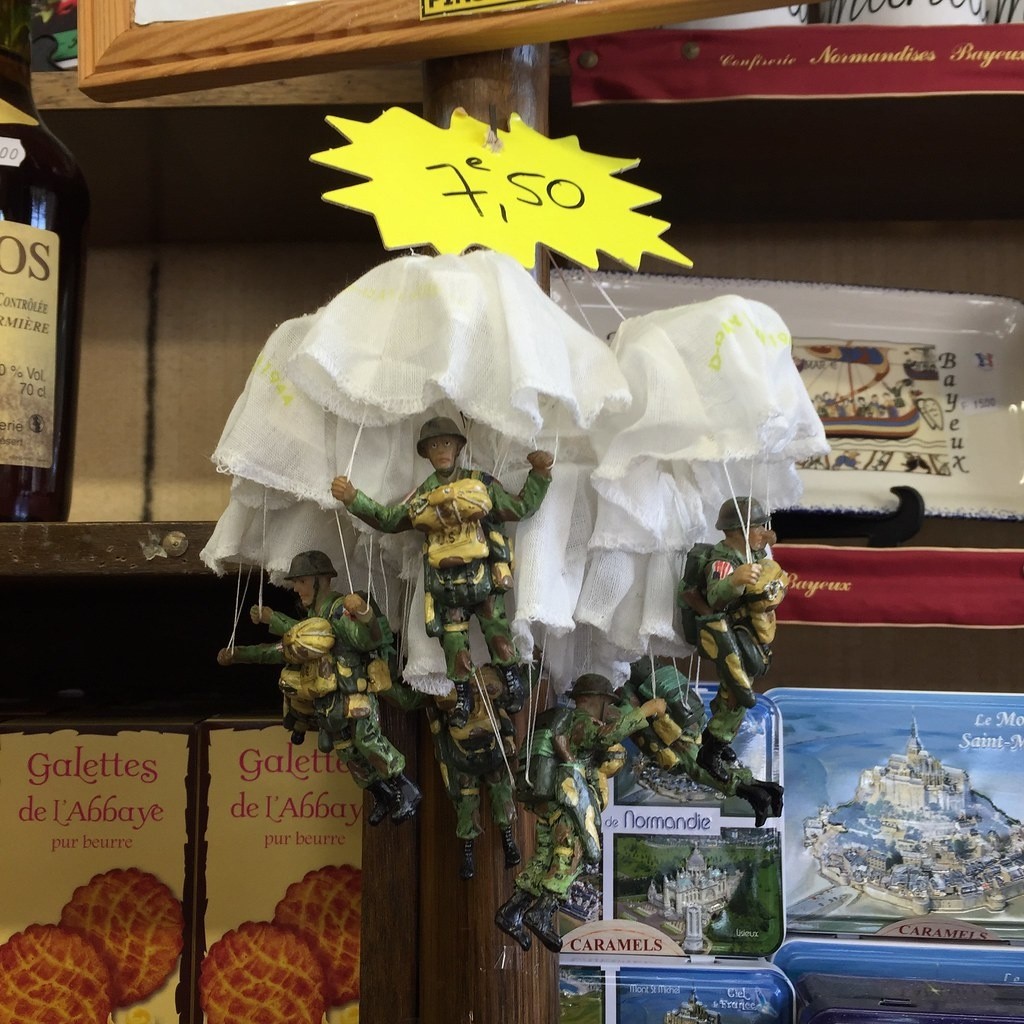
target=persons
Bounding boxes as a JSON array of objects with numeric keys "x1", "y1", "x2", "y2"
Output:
[
  {"x1": 333, "y1": 415, "x2": 552, "y2": 726},
  {"x1": 373, "y1": 649, "x2": 525, "y2": 880},
  {"x1": 612, "y1": 653, "x2": 786, "y2": 829},
  {"x1": 691, "y1": 494, "x2": 782, "y2": 780},
  {"x1": 217, "y1": 548, "x2": 423, "y2": 823},
  {"x1": 496, "y1": 674, "x2": 672, "y2": 954}
]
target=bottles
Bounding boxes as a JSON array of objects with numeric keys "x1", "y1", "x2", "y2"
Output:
[{"x1": 0, "y1": 0, "x2": 88, "y2": 523}]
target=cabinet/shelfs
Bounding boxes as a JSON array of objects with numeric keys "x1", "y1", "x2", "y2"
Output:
[{"x1": 0, "y1": 23, "x2": 1024, "y2": 630}]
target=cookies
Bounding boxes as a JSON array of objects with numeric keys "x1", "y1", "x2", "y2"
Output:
[
  {"x1": 192, "y1": 862, "x2": 363, "y2": 1022},
  {"x1": 0, "y1": 867, "x2": 184, "y2": 1024}
]
